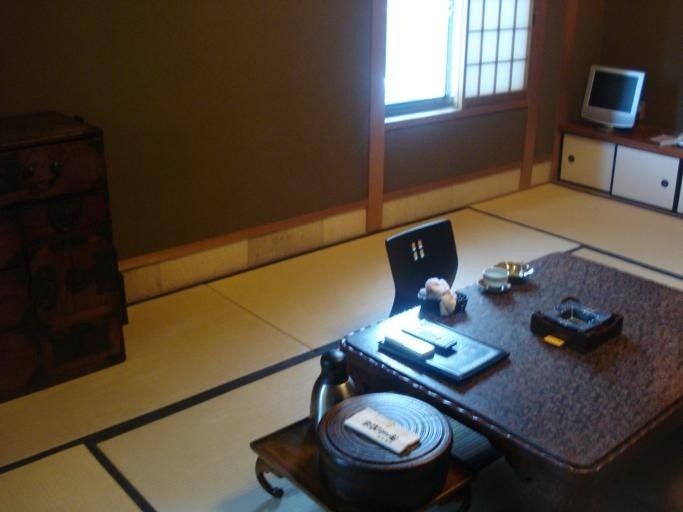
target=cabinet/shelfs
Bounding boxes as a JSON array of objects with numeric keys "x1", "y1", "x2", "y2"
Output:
[
  {"x1": 1, "y1": 110, "x2": 129, "y2": 403},
  {"x1": 550, "y1": 118, "x2": 683, "y2": 219}
]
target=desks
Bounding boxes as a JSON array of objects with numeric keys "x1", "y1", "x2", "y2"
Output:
[{"x1": 249, "y1": 415, "x2": 481, "y2": 512}]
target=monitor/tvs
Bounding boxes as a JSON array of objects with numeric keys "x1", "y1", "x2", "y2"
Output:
[{"x1": 581, "y1": 65, "x2": 645, "y2": 129}]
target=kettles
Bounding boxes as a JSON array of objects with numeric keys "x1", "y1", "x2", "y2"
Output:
[{"x1": 309, "y1": 349, "x2": 361, "y2": 435}]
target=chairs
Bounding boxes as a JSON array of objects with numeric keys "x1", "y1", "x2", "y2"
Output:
[{"x1": 384, "y1": 219, "x2": 460, "y2": 317}]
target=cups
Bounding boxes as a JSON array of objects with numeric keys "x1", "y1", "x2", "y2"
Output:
[{"x1": 483, "y1": 267, "x2": 509, "y2": 288}]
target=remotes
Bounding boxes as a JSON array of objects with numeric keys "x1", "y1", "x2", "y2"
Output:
[
  {"x1": 402, "y1": 323, "x2": 457, "y2": 350},
  {"x1": 384, "y1": 330, "x2": 435, "y2": 358}
]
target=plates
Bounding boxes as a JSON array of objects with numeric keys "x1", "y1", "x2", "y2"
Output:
[
  {"x1": 495, "y1": 261, "x2": 535, "y2": 283},
  {"x1": 476, "y1": 279, "x2": 513, "y2": 293}
]
